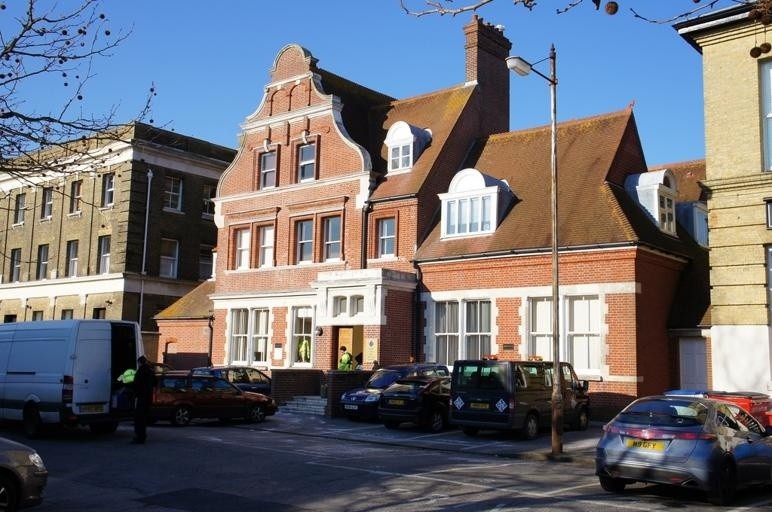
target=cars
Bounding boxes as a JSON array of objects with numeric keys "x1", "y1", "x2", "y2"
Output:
[
  {"x1": 594, "y1": 394, "x2": 772, "y2": 507},
  {"x1": 0, "y1": 436, "x2": 49, "y2": 511},
  {"x1": 151, "y1": 366, "x2": 280, "y2": 427},
  {"x1": 338, "y1": 362, "x2": 451, "y2": 435}
]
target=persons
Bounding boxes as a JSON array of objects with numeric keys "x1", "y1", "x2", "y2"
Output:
[
  {"x1": 131, "y1": 354, "x2": 155, "y2": 446},
  {"x1": 336, "y1": 346, "x2": 353, "y2": 371},
  {"x1": 410, "y1": 355, "x2": 417, "y2": 363}
]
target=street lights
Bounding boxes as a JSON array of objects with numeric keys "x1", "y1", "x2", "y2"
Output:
[{"x1": 505, "y1": 41, "x2": 563, "y2": 459}]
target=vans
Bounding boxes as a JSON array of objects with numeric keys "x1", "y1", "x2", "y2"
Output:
[{"x1": 448, "y1": 354, "x2": 590, "y2": 440}]
